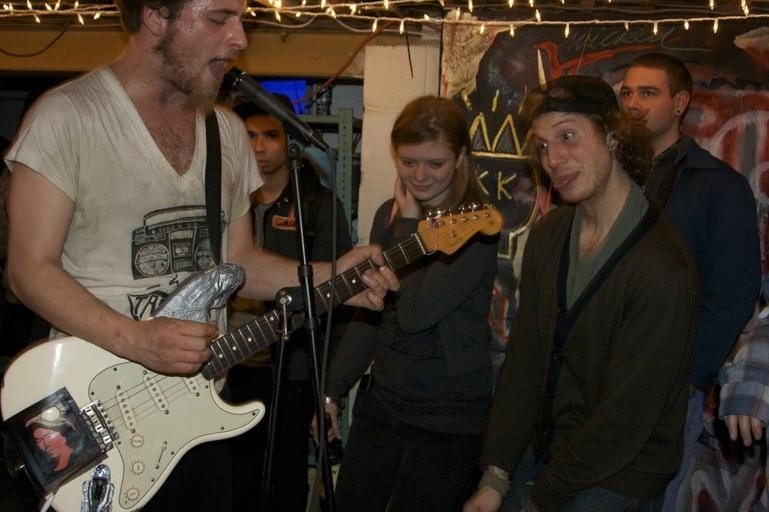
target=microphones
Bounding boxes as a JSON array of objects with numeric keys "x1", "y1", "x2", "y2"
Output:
[{"x1": 225, "y1": 66, "x2": 329, "y2": 151}]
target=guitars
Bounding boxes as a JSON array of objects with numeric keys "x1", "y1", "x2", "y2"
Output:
[{"x1": 0, "y1": 203, "x2": 504, "y2": 512}]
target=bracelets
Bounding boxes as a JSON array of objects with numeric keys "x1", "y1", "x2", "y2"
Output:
[
  {"x1": 477, "y1": 471, "x2": 511, "y2": 495},
  {"x1": 324, "y1": 396, "x2": 337, "y2": 404}
]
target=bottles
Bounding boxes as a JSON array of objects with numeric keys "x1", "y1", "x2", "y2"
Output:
[{"x1": 315, "y1": 412, "x2": 343, "y2": 511}]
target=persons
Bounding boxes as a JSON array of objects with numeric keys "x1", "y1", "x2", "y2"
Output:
[
  {"x1": 620, "y1": 54, "x2": 763, "y2": 512},
  {"x1": 217, "y1": 93, "x2": 351, "y2": 512},
  {"x1": 1, "y1": 1, "x2": 399, "y2": 512},
  {"x1": 718, "y1": 304, "x2": 768, "y2": 512},
  {"x1": 312, "y1": 95, "x2": 499, "y2": 512},
  {"x1": 462, "y1": 74, "x2": 696, "y2": 512}
]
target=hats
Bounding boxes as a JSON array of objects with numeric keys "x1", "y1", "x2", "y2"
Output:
[{"x1": 518, "y1": 75, "x2": 620, "y2": 131}]
